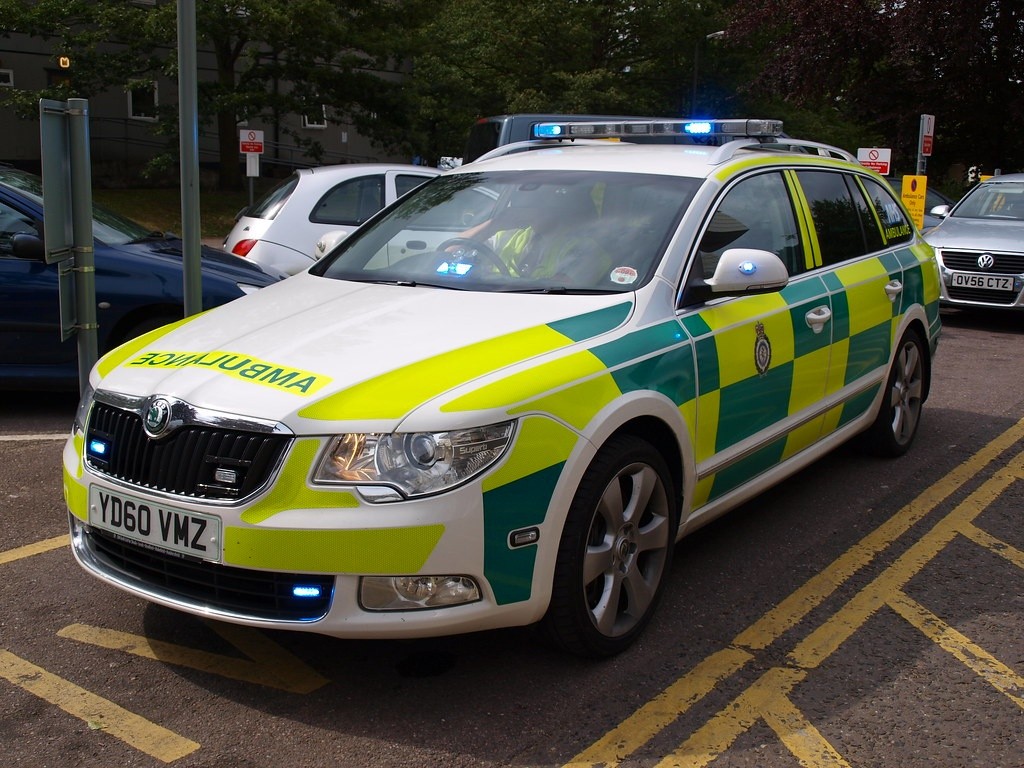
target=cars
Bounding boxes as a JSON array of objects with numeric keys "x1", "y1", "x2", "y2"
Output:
[
  {"x1": 221, "y1": 161, "x2": 511, "y2": 284},
  {"x1": 62, "y1": 109, "x2": 944, "y2": 660},
  {"x1": 921, "y1": 173, "x2": 1024, "y2": 313},
  {"x1": 884, "y1": 176, "x2": 958, "y2": 236},
  {"x1": 0, "y1": 166, "x2": 284, "y2": 395}
]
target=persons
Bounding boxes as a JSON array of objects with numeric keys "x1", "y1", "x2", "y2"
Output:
[
  {"x1": 444, "y1": 190, "x2": 603, "y2": 291},
  {"x1": 968, "y1": 162, "x2": 982, "y2": 185}
]
